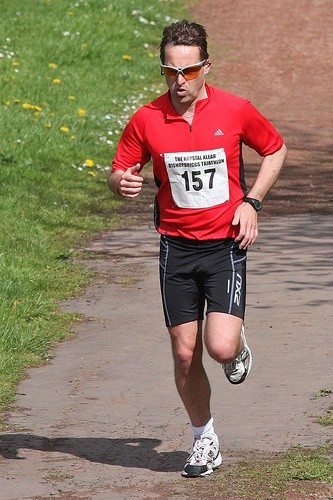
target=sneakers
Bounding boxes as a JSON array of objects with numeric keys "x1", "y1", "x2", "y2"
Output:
[
  {"x1": 181, "y1": 433, "x2": 222, "y2": 477},
  {"x1": 221, "y1": 322, "x2": 253, "y2": 386}
]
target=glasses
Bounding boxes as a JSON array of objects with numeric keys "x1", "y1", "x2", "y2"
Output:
[{"x1": 160, "y1": 59, "x2": 207, "y2": 80}]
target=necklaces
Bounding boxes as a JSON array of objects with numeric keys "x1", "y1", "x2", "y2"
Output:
[{"x1": 173, "y1": 105, "x2": 195, "y2": 118}]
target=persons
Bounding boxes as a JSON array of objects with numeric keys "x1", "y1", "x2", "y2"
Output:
[{"x1": 108, "y1": 19, "x2": 288, "y2": 478}]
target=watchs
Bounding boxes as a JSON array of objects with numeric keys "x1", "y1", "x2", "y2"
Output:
[{"x1": 243, "y1": 197, "x2": 263, "y2": 212}]
